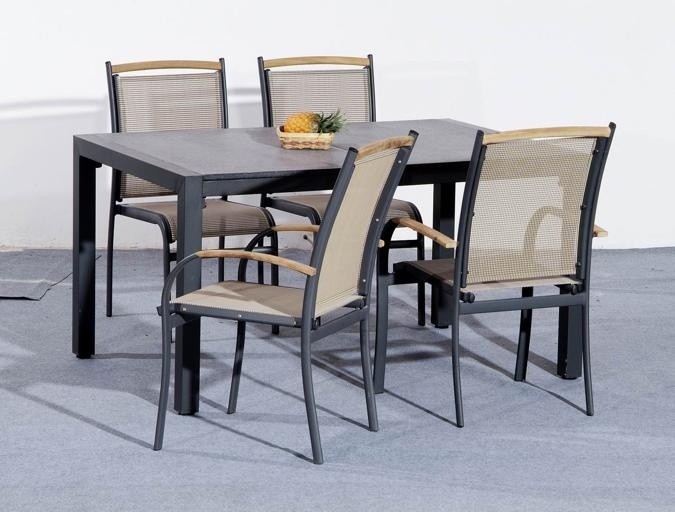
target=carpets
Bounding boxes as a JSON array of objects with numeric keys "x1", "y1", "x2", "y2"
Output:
[{"x1": 0, "y1": 248, "x2": 102, "y2": 301}]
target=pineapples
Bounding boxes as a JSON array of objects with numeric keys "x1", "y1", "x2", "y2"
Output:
[{"x1": 283, "y1": 108, "x2": 347, "y2": 136}]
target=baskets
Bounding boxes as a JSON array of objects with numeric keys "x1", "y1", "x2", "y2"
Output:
[{"x1": 276, "y1": 125, "x2": 334, "y2": 150}]
